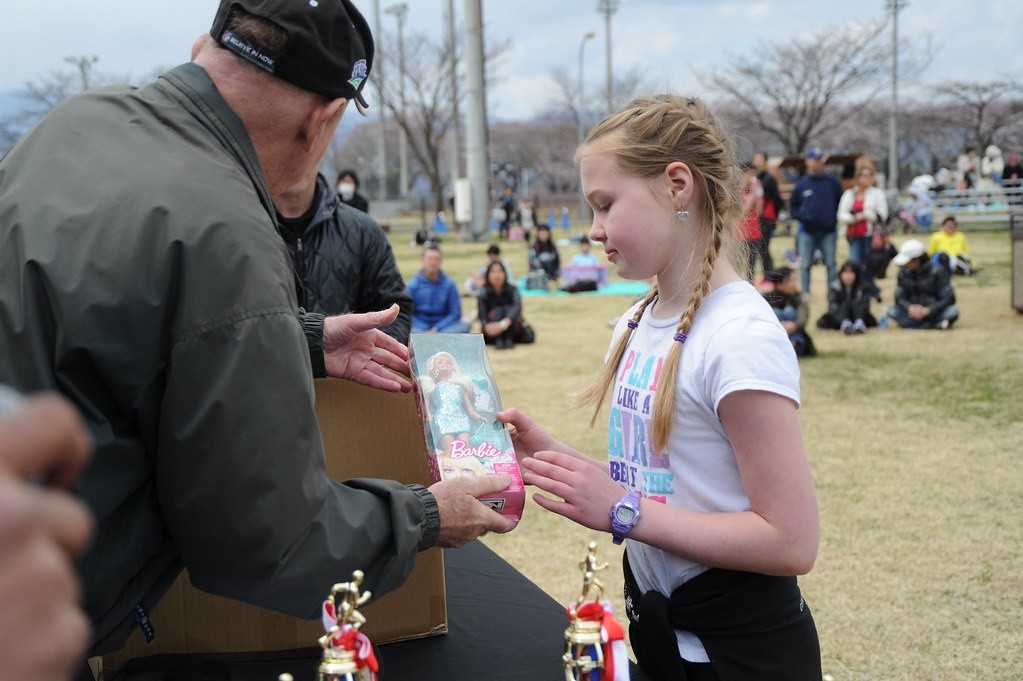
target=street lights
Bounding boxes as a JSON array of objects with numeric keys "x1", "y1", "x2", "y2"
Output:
[
  {"x1": 597, "y1": 0, "x2": 622, "y2": 114},
  {"x1": 577, "y1": 31, "x2": 595, "y2": 225},
  {"x1": 886, "y1": 0, "x2": 909, "y2": 189},
  {"x1": 383, "y1": 2, "x2": 409, "y2": 198}
]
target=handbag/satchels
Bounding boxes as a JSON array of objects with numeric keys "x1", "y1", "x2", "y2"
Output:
[
  {"x1": 514, "y1": 326, "x2": 534, "y2": 343},
  {"x1": 870, "y1": 223, "x2": 891, "y2": 251}
]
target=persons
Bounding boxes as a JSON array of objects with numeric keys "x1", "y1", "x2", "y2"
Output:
[
  {"x1": 320, "y1": 570, "x2": 371, "y2": 659},
  {"x1": 496, "y1": 93, "x2": 826, "y2": 681},
  {"x1": 407, "y1": 186, "x2": 603, "y2": 351},
  {"x1": 0, "y1": 0, "x2": 519, "y2": 681},
  {"x1": 335, "y1": 169, "x2": 369, "y2": 213},
  {"x1": 572, "y1": 542, "x2": 609, "y2": 611},
  {"x1": 269, "y1": 165, "x2": 412, "y2": 345},
  {"x1": 0, "y1": 388, "x2": 97, "y2": 681},
  {"x1": 728, "y1": 144, "x2": 1023, "y2": 358}
]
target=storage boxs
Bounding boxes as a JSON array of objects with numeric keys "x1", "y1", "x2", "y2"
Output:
[{"x1": 104, "y1": 365, "x2": 448, "y2": 678}]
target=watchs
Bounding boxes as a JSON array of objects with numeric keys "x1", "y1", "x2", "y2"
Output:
[{"x1": 609, "y1": 491, "x2": 641, "y2": 545}]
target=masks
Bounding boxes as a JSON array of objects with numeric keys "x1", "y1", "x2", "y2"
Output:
[{"x1": 337, "y1": 183, "x2": 356, "y2": 195}]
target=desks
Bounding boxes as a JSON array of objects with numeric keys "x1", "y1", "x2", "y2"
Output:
[{"x1": 112, "y1": 539, "x2": 655, "y2": 681}]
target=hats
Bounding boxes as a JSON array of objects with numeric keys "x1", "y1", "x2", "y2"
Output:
[
  {"x1": 769, "y1": 266, "x2": 794, "y2": 282},
  {"x1": 210, "y1": 0, "x2": 375, "y2": 118},
  {"x1": 806, "y1": 148, "x2": 825, "y2": 159},
  {"x1": 891, "y1": 240, "x2": 924, "y2": 266}
]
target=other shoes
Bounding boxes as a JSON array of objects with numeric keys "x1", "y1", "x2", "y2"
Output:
[
  {"x1": 840, "y1": 318, "x2": 854, "y2": 334},
  {"x1": 936, "y1": 318, "x2": 950, "y2": 329},
  {"x1": 855, "y1": 318, "x2": 867, "y2": 333},
  {"x1": 496, "y1": 338, "x2": 515, "y2": 349}
]
